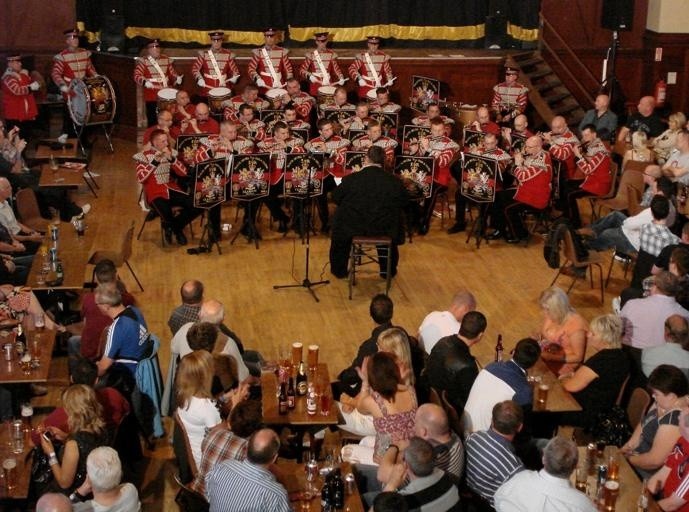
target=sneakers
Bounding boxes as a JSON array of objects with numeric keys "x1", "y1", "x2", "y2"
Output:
[
  {"x1": 614, "y1": 251, "x2": 631, "y2": 263},
  {"x1": 77, "y1": 203, "x2": 91, "y2": 219}
]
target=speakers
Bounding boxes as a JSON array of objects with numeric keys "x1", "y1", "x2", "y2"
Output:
[{"x1": 601, "y1": 0, "x2": 634, "y2": 32}]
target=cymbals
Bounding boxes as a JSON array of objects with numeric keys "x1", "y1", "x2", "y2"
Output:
[{"x1": 31, "y1": 70, "x2": 46, "y2": 101}]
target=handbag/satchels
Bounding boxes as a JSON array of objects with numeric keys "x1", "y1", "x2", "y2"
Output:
[
  {"x1": 25, "y1": 440, "x2": 63, "y2": 489},
  {"x1": 583, "y1": 406, "x2": 632, "y2": 446}
]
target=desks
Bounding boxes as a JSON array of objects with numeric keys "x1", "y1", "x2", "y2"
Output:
[
  {"x1": 240, "y1": 347, "x2": 664, "y2": 511},
  {"x1": 0, "y1": 84, "x2": 99, "y2": 494}
]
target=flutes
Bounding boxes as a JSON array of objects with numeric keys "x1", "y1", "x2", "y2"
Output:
[{"x1": 153, "y1": 104, "x2": 591, "y2": 165}]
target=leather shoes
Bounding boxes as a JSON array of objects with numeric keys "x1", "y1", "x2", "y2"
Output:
[
  {"x1": 505, "y1": 228, "x2": 520, "y2": 243},
  {"x1": 487, "y1": 228, "x2": 501, "y2": 240},
  {"x1": 163, "y1": 214, "x2": 290, "y2": 246},
  {"x1": 447, "y1": 221, "x2": 466, "y2": 234}
]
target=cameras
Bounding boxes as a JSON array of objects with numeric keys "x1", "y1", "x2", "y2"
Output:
[{"x1": 43, "y1": 430, "x2": 52, "y2": 441}]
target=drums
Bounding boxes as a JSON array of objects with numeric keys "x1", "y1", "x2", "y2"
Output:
[
  {"x1": 266, "y1": 89, "x2": 288, "y2": 110},
  {"x1": 208, "y1": 87, "x2": 231, "y2": 115},
  {"x1": 68, "y1": 77, "x2": 116, "y2": 126},
  {"x1": 157, "y1": 88, "x2": 178, "y2": 114},
  {"x1": 318, "y1": 86, "x2": 336, "y2": 105},
  {"x1": 367, "y1": 89, "x2": 388, "y2": 102}
]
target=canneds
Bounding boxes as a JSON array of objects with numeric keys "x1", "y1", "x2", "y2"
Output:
[
  {"x1": 599, "y1": 465, "x2": 607, "y2": 485},
  {"x1": 52, "y1": 241, "x2": 57, "y2": 248},
  {"x1": 221, "y1": 222, "x2": 232, "y2": 232},
  {"x1": 50, "y1": 248, "x2": 56, "y2": 262},
  {"x1": 52, "y1": 227, "x2": 58, "y2": 241},
  {"x1": 13, "y1": 439, "x2": 24, "y2": 454},
  {"x1": 586, "y1": 443, "x2": 598, "y2": 465},
  {"x1": 52, "y1": 262, "x2": 56, "y2": 272},
  {"x1": 13, "y1": 420, "x2": 23, "y2": 439},
  {"x1": 4, "y1": 343, "x2": 13, "y2": 361},
  {"x1": 345, "y1": 473, "x2": 356, "y2": 495},
  {"x1": 5, "y1": 361, "x2": 13, "y2": 373},
  {"x1": 279, "y1": 366, "x2": 289, "y2": 385},
  {"x1": 304, "y1": 460, "x2": 318, "y2": 482}
]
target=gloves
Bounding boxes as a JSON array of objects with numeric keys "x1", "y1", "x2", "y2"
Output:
[
  {"x1": 339, "y1": 78, "x2": 344, "y2": 85},
  {"x1": 197, "y1": 79, "x2": 205, "y2": 88},
  {"x1": 387, "y1": 80, "x2": 393, "y2": 85},
  {"x1": 144, "y1": 81, "x2": 155, "y2": 88},
  {"x1": 256, "y1": 78, "x2": 265, "y2": 87},
  {"x1": 358, "y1": 79, "x2": 366, "y2": 87},
  {"x1": 60, "y1": 85, "x2": 69, "y2": 92},
  {"x1": 176, "y1": 76, "x2": 183, "y2": 84},
  {"x1": 309, "y1": 75, "x2": 317, "y2": 84},
  {"x1": 230, "y1": 76, "x2": 237, "y2": 84},
  {"x1": 30, "y1": 81, "x2": 40, "y2": 91}
]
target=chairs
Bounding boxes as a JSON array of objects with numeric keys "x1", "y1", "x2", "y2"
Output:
[
  {"x1": 169, "y1": 410, "x2": 210, "y2": 511},
  {"x1": 87, "y1": 221, "x2": 146, "y2": 292},
  {"x1": 136, "y1": 100, "x2": 689, "y2": 314}
]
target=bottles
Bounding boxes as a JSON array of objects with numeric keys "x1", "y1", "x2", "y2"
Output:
[
  {"x1": 274, "y1": 341, "x2": 333, "y2": 416},
  {"x1": 495, "y1": 333, "x2": 504, "y2": 363},
  {"x1": 37, "y1": 220, "x2": 64, "y2": 285},
  {"x1": 571, "y1": 440, "x2": 621, "y2": 511},
  {"x1": 74, "y1": 212, "x2": 85, "y2": 235},
  {"x1": 636, "y1": 478, "x2": 651, "y2": 511},
  {"x1": 295, "y1": 448, "x2": 356, "y2": 511}
]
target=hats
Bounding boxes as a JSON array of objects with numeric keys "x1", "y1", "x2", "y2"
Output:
[
  {"x1": 208, "y1": 32, "x2": 225, "y2": 40},
  {"x1": 147, "y1": 39, "x2": 161, "y2": 48},
  {"x1": 264, "y1": 28, "x2": 277, "y2": 36},
  {"x1": 367, "y1": 36, "x2": 381, "y2": 44},
  {"x1": 6, "y1": 53, "x2": 25, "y2": 61},
  {"x1": 64, "y1": 28, "x2": 82, "y2": 37},
  {"x1": 504, "y1": 67, "x2": 519, "y2": 75},
  {"x1": 314, "y1": 31, "x2": 329, "y2": 42}
]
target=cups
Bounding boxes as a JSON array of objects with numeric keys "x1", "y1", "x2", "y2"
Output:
[
  {"x1": 21, "y1": 429, "x2": 32, "y2": 448},
  {"x1": 0, "y1": 309, "x2": 46, "y2": 374},
  {"x1": 0, "y1": 458, "x2": 18, "y2": 490},
  {"x1": 538, "y1": 386, "x2": 549, "y2": 403},
  {"x1": 49, "y1": 159, "x2": 58, "y2": 172},
  {"x1": 527, "y1": 370, "x2": 543, "y2": 390},
  {"x1": 7, "y1": 420, "x2": 24, "y2": 454}
]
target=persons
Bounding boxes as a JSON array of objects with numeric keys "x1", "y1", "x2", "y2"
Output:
[
  {"x1": 0, "y1": 52, "x2": 42, "y2": 145},
  {"x1": 31, "y1": 281, "x2": 152, "y2": 512},
  {"x1": 549, "y1": 84, "x2": 688, "y2": 509},
  {"x1": 51, "y1": 29, "x2": 102, "y2": 142},
  {"x1": 166, "y1": 281, "x2": 289, "y2": 512},
  {"x1": 329, "y1": 290, "x2": 541, "y2": 512},
  {"x1": 133, "y1": 24, "x2": 546, "y2": 243},
  {"x1": 326, "y1": 144, "x2": 408, "y2": 279},
  {"x1": 0, "y1": 121, "x2": 90, "y2": 347}
]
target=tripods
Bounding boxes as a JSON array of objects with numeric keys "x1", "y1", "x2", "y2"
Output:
[
  {"x1": 274, "y1": 161, "x2": 330, "y2": 303},
  {"x1": 199, "y1": 210, "x2": 221, "y2": 256},
  {"x1": 466, "y1": 203, "x2": 489, "y2": 250},
  {"x1": 283, "y1": 200, "x2": 317, "y2": 244},
  {"x1": 349, "y1": 245, "x2": 380, "y2": 278},
  {"x1": 231, "y1": 200, "x2": 262, "y2": 250}
]
